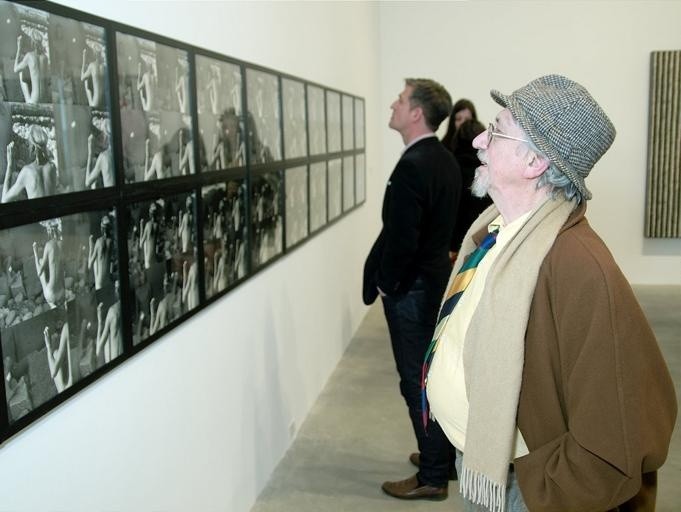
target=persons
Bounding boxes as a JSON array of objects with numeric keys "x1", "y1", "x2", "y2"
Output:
[
  {"x1": 419, "y1": 73, "x2": 678, "y2": 512},
  {"x1": 33, "y1": 215, "x2": 123, "y2": 394},
  {"x1": 2, "y1": 31, "x2": 115, "y2": 205},
  {"x1": 137, "y1": 59, "x2": 280, "y2": 182},
  {"x1": 438, "y1": 96, "x2": 479, "y2": 153},
  {"x1": 139, "y1": 184, "x2": 281, "y2": 336},
  {"x1": 360, "y1": 75, "x2": 461, "y2": 503},
  {"x1": 450, "y1": 119, "x2": 494, "y2": 252}
]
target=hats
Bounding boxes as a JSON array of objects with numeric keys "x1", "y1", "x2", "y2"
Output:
[{"x1": 489, "y1": 73, "x2": 617, "y2": 202}]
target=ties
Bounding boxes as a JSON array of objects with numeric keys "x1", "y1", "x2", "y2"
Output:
[{"x1": 421, "y1": 229, "x2": 499, "y2": 439}]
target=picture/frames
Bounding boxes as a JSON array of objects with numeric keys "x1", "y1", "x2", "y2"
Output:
[{"x1": 0, "y1": 0, "x2": 366, "y2": 442}]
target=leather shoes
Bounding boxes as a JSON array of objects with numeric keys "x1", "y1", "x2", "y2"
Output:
[
  {"x1": 409, "y1": 453, "x2": 422, "y2": 467},
  {"x1": 381, "y1": 473, "x2": 449, "y2": 502}
]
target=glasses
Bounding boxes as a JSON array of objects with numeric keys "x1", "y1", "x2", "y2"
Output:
[{"x1": 487, "y1": 122, "x2": 534, "y2": 145}]
